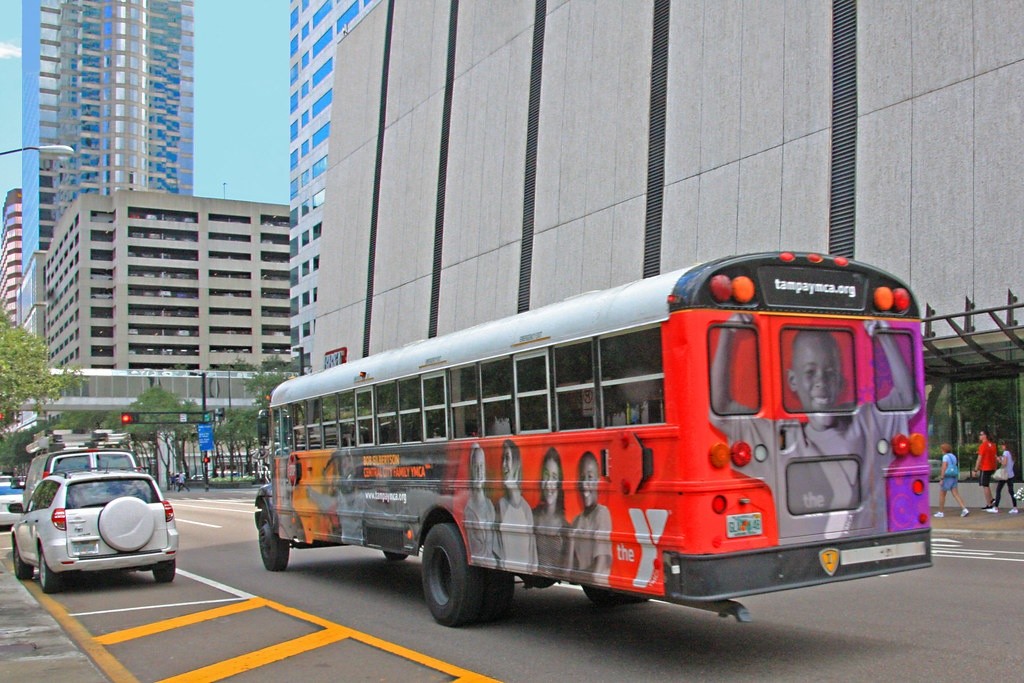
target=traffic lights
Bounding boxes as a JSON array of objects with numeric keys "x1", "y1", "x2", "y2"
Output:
[{"x1": 121, "y1": 412, "x2": 139, "y2": 425}]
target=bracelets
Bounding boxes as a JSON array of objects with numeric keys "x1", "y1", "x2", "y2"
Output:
[
  {"x1": 974, "y1": 470, "x2": 977, "y2": 472},
  {"x1": 999, "y1": 460, "x2": 1001, "y2": 463},
  {"x1": 940, "y1": 478, "x2": 944, "y2": 480}
]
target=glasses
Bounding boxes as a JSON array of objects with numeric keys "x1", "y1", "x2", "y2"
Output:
[{"x1": 979, "y1": 433, "x2": 984, "y2": 436}]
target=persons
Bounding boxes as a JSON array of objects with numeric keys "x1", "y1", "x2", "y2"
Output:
[
  {"x1": 169, "y1": 472, "x2": 189, "y2": 491},
  {"x1": 497, "y1": 439, "x2": 538, "y2": 574},
  {"x1": 464, "y1": 447, "x2": 497, "y2": 568},
  {"x1": 573, "y1": 452, "x2": 613, "y2": 586},
  {"x1": 534, "y1": 447, "x2": 574, "y2": 579},
  {"x1": 306, "y1": 448, "x2": 410, "y2": 546},
  {"x1": 708, "y1": 313, "x2": 921, "y2": 545},
  {"x1": 933, "y1": 444, "x2": 970, "y2": 518},
  {"x1": 973, "y1": 430, "x2": 998, "y2": 510},
  {"x1": 987, "y1": 441, "x2": 1019, "y2": 514}
]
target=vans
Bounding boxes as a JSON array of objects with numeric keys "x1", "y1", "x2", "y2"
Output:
[{"x1": 22, "y1": 429, "x2": 142, "y2": 512}]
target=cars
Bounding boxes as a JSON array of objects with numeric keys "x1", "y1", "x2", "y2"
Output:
[{"x1": 0, "y1": 481, "x2": 24, "y2": 530}]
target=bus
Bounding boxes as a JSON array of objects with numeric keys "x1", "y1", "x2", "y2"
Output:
[{"x1": 254, "y1": 251, "x2": 933, "y2": 627}]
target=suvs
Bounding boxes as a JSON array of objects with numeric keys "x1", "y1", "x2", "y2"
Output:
[{"x1": 11, "y1": 462, "x2": 179, "y2": 594}]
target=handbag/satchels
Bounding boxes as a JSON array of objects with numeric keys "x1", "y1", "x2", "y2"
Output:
[
  {"x1": 944, "y1": 454, "x2": 957, "y2": 477},
  {"x1": 991, "y1": 464, "x2": 1008, "y2": 480}
]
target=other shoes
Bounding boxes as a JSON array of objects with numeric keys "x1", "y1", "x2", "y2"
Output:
[
  {"x1": 987, "y1": 507, "x2": 998, "y2": 513},
  {"x1": 981, "y1": 505, "x2": 992, "y2": 511},
  {"x1": 991, "y1": 499, "x2": 995, "y2": 504}
]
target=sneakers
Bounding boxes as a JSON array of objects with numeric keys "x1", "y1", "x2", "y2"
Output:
[
  {"x1": 1008, "y1": 509, "x2": 1018, "y2": 513},
  {"x1": 933, "y1": 512, "x2": 944, "y2": 517},
  {"x1": 960, "y1": 509, "x2": 969, "y2": 516}
]
